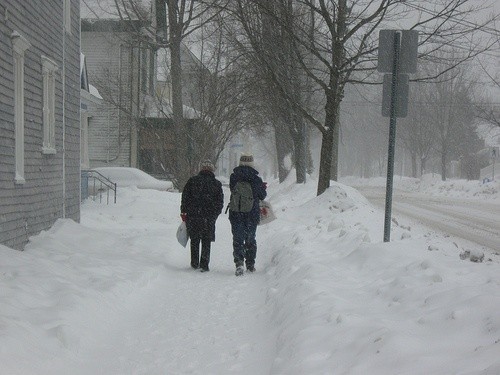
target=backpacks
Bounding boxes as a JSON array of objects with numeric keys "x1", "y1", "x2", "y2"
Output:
[{"x1": 224, "y1": 173, "x2": 257, "y2": 215}]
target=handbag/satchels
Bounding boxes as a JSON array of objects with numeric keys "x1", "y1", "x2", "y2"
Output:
[
  {"x1": 258, "y1": 198, "x2": 277, "y2": 226},
  {"x1": 176, "y1": 221, "x2": 189, "y2": 248}
]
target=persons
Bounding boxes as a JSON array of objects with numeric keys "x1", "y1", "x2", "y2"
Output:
[
  {"x1": 229, "y1": 154, "x2": 267, "y2": 276},
  {"x1": 180, "y1": 159, "x2": 223, "y2": 271}
]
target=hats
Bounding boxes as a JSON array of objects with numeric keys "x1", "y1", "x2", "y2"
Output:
[{"x1": 239, "y1": 153, "x2": 255, "y2": 168}]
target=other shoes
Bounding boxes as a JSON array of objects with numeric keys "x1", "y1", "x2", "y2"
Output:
[
  {"x1": 235, "y1": 266, "x2": 244, "y2": 276},
  {"x1": 247, "y1": 267, "x2": 256, "y2": 273}
]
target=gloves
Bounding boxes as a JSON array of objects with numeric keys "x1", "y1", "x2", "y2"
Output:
[{"x1": 180, "y1": 211, "x2": 188, "y2": 222}]
